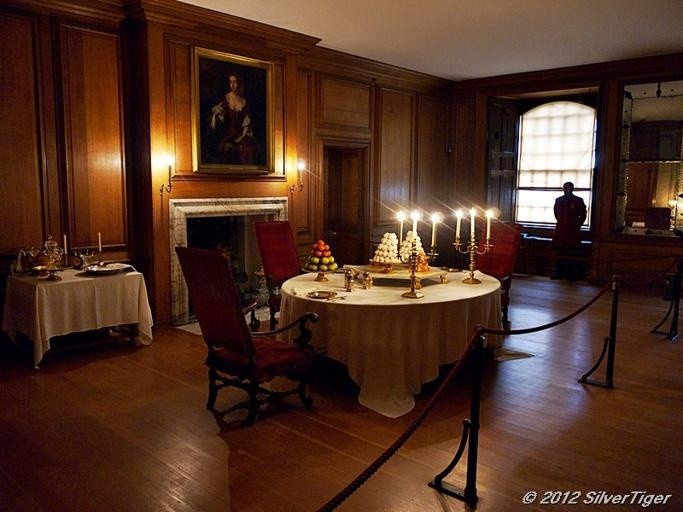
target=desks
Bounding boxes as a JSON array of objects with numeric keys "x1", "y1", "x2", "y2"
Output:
[{"x1": 3, "y1": 264, "x2": 154, "y2": 365}]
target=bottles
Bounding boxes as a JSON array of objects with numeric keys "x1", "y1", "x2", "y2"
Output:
[{"x1": 343, "y1": 268, "x2": 373, "y2": 292}]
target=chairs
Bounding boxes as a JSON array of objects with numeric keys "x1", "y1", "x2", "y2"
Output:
[
  {"x1": 174, "y1": 247, "x2": 321, "y2": 426},
  {"x1": 467, "y1": 217, "x2": 523, "y2": 333},
  {"x1": 252, "y1": 221, "x2": 304, "y2": 332}
]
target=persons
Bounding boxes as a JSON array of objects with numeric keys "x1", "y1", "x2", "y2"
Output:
[
  {"x1": 548, "y1": 181, "x2": 587, "y2": 280},
  {"x1": 204, "y1": 69, "x2": 257, "y2": 163}
]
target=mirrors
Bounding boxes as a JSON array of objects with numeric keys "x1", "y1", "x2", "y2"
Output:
[{"x1": 607, "y1": 72, "x2": 683, "y2": 246}]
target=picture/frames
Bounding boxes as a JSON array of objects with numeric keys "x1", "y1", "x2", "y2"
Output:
[{"x1": 190, "y1": 46, "x2": 278, "y2": 175}]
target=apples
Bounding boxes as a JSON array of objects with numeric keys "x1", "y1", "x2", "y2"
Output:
[{"x1": 304, "y1": 240, "x2": 339, "y2": 270}]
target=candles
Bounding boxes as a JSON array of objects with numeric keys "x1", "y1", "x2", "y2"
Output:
[
  {"x1": 63, "y1": 235, "x2": 67, "y2": 252},
  {"x1": 97, "y1": 231, "x2": 102, "y2": 252}
]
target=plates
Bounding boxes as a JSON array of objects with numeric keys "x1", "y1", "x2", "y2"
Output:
[
  {"x1": 87, "y1": 266, "x2": 123, "y2": 275},
  {"x1": 305, "y1": 289, "x2": 338, "y2": 300}
]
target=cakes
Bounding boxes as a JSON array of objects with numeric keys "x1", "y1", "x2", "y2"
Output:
[
  {"x1": 373, "y1": 232, "x2": 400, "y2": 262},
  {"x1": 401, "y1": 230, "x2": 426, "y2": 263}
]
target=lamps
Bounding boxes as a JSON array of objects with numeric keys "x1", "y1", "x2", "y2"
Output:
[
  {"x1": 395, "y1": 207, "x2": 439, "y2": 301},
  {"x1": 452, "y1": 205, "x2": 494, "y2": 285}
]
